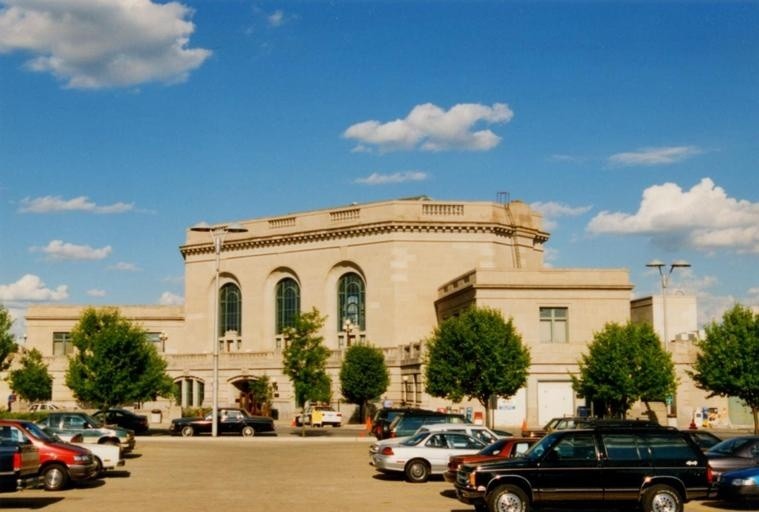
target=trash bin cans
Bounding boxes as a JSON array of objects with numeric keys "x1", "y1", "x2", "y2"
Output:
[
  {"x1": 667, "y1": 413, "x2": 677, "y2": 428},
  {"x1": 151, "y1": 409, "x2": 162, "y2": 424},
  {"x1": 272, "y1": 409, "x2": 278, "y2": 420}
]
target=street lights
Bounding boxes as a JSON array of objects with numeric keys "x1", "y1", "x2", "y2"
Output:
[
  {"x1": 646, "y1": 260, "x2": 691, "y2": 414},
  {"x1": 191, "y1": 221, "x2": 248, "y2": 437}
]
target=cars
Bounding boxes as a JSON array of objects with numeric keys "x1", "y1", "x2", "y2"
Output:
[
  {"x1": 372, "y1": 430, "x2": 498, "y2": 483},
  {"x1": 296, "y1": 406, "x2": 342, "y2": 427},
  {"x1": 701, "y1": 436, "x2": 758, "y2": 483},
  {"x1": 0, "y1": 403, "x2": 149, "y2": 491},
  {"x1": 715, "y1": 467, "x2": 758, "y2": 508},
  {"x1": 442, "y1": 436, "x2": 541, "y2": 484},
  {"x1": 169, "y1": 408, "x2": 275, "y2": 437},
  {"x1": 683, "y1": 429, "x2": 722, "y2": 453},
  {"x1": 368, "y1": 408, "x2": 514, "y2": 458}
]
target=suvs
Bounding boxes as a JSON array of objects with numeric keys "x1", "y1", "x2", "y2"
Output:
[
  {"x1": 454, "y1": 423, "x2": 715, "y2": 512},
  {"x1": 521, "y1": 418, "x2": 588, "y2": 439}
]
